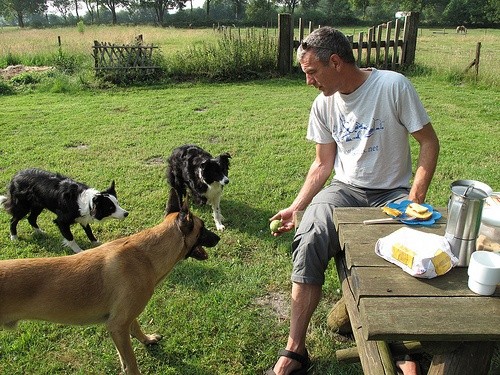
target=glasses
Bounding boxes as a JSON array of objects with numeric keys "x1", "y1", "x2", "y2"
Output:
[{"x1": 300, "y1": 39, "x2": 336, "y2": 52}]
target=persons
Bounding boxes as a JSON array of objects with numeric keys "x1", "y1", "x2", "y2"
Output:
[{"x1": 263, "y1": 26, "x2": 440, "y2": 375}]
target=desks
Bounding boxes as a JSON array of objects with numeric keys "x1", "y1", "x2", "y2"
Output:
[{"x1": 332, "y1": 207, "x2": 500, "y2": 375}]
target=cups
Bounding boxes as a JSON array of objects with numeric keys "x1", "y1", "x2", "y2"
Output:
[{"x1": 467, "y1": 250, "x2": 500, "y2": 294}]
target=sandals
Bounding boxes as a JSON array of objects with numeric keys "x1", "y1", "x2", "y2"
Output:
[{"x1": 264, "y1": 347, "x2": 316, "y2": 374}]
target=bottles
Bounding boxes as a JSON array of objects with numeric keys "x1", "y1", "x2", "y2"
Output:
[{"x1": 475, "y1": 194, "x2": 499, "y2": 253}]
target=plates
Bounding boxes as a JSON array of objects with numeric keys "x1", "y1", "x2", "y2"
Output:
[{"x1": 386, "y1": 200, "x2": 443, "y2": 226}]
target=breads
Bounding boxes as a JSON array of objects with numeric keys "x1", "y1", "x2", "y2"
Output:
[
  {"x1": 475, "y1": 235, "x2": 500, "y2": 253},
  {"x1": 380, "y1": 206, "x2": 402, "y2": 217},
  {"x1": 405, "y1": 203, "x2": 433, "y2": 220}
]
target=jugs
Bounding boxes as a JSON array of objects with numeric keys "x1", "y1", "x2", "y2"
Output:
[{"x1": 443, "y1": 179, "x2": 492, "y2": 267}]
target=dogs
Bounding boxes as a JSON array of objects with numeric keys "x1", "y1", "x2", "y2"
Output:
[
  {"x1": 0, "y1": 168, "x2": 130, "y2": 253},
  {"x1": 0, "y1": 193, "x2": 220, "y2": 375},
  {"x1": 166, "y1": 144, "x2": 233, "y2": 232}
]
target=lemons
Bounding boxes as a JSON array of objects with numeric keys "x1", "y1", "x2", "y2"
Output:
[{"x1": 269, "y1": 220, "x2": 283, "y2": 233}]
